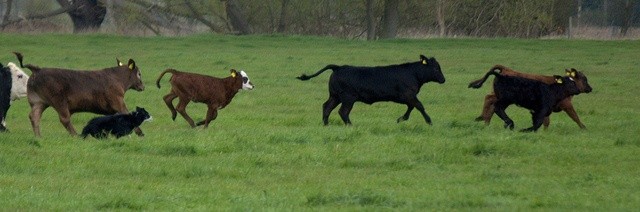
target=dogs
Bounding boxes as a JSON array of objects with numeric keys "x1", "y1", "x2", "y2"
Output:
[{"x1": 79, "y1": 106, "x2": 153, "y2": 141}]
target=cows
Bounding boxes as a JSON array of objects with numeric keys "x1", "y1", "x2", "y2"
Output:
[
  {"x1": 0, "y1": 61, "x2": 29, "y2": 133},
  {"x1": 156, "y1": 69, "x2": 254, "y2": 128},
  {"x1": 468, "y1": 68, "x2": 592, "y2": 130},
  {"x1": 295, "y1": 54, "x2": 445, "y2": 126},
  {"x1": 472, "y1": 71, "x2": 580, "y2": 132},
  {"x1": 11, "y1": 51, "x2": 145, "y2": 138}
]
